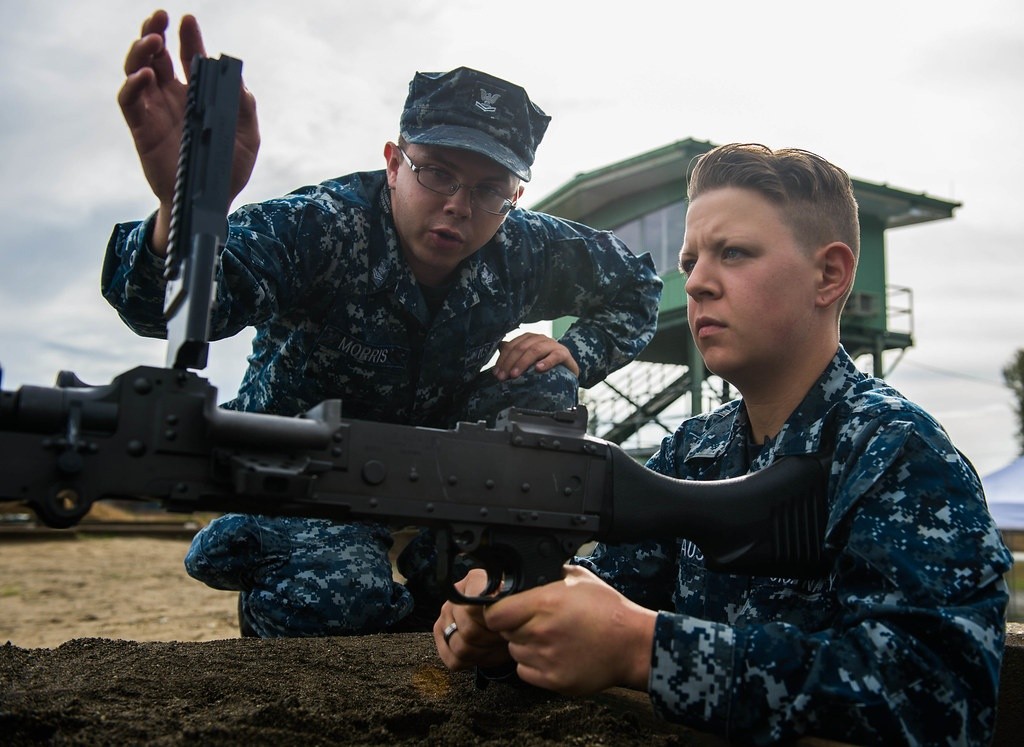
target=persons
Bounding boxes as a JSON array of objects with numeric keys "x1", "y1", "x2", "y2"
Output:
[
  {"x1": 99, "y1": 10, "x2": 665, "y2": 639},
  {"x1": 431, "y1": 143, "x2": 1016, "y2": 746}
]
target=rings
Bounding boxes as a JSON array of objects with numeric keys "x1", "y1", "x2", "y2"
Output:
[{"x1": 443, "y1": 621, "x2": 457, "y2": 644}]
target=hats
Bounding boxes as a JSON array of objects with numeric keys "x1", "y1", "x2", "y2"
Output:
[{"x1": 398, "y1": 66, "x2": 552, "y2": 185}]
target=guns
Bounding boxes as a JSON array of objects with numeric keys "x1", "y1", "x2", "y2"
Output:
[
  {"x1": 1, "y1": 365, "x2": 842, "y2": 694},
  {"x1": 162, "y1": 53, "x2": 246, "y2": 372}
]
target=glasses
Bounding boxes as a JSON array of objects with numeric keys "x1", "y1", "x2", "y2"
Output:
[{"x1": 393, "y1": 145, "x2": 519, "y2": 218}]
